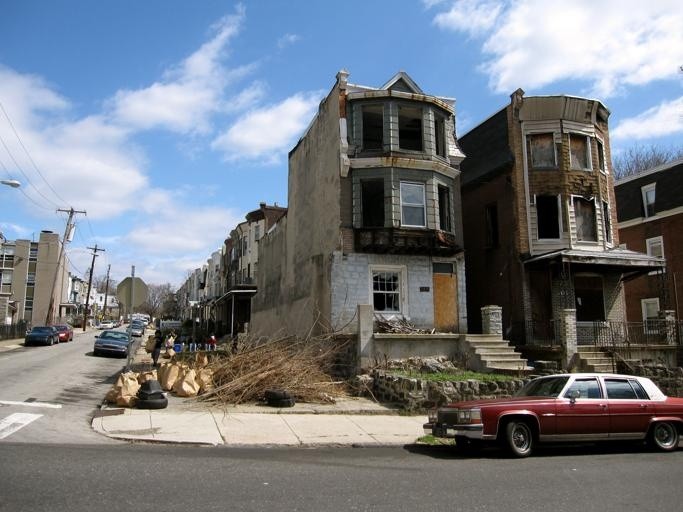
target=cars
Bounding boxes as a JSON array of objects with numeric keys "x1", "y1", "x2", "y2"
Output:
[
  {"x1": 423, "y1": 372, "x2": 682, "y2": 460},
  {"x1": 88, "y1": 311, "x2": 150, "y2": 358},
  {"x1": 26, "y1": 322, "x2": 74, "y2": 347}
]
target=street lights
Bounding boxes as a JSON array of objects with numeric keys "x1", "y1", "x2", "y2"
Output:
[{"x1": 0, "y1": 180, "x2": 21, "y2": 188}]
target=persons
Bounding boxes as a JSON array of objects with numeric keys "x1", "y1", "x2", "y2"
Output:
[
  {"x1": 149, "y1": 330, "x2": 162, "y2": 366},
  {"x1": 165, "y1": 328, "x2": 178, "y2": 355}
]
